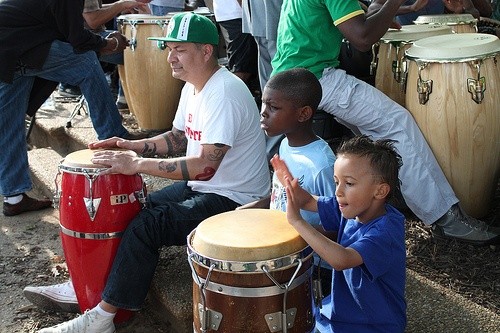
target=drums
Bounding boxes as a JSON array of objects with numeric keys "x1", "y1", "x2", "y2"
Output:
[
  {"x1": 123, "y1": 16, "x2": 186, "y2": 132},
  {"x1": 57, "y1": 147, "x2": 147, "y2": 330},
  {"x1": 187, "y1": 207, "x2": 317, "y2": 333},
  {"x1": 414, "y1": 13, "x2": 479, "y2": 34},
  {"x1": 398, "y1": 32, "x2": 500, "y2": 223},
  {"x1": 374, "y1": 23, "x2": 453, "y2": 109},
  {"x1": 115, "y1": 13, "x2": 159, "y2": 121}
]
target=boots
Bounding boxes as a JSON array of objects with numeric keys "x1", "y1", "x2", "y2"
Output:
[{"x1": 435, "y1": 202, "x2": 500, "y2": 247}]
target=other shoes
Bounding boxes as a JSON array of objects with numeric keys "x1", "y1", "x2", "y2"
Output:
[
  {"x1": 116, "y1": 101, "x2": 129, "y2": 112},
  {"x1": 58, "y1": 85, "x2": 81, "y2": 97},
  {"x1": 105, "y1": 131, "x2": 150, "y2": 140},
  {"x1": 3, "y1": 192, "x2": 52, "y2": 217}
]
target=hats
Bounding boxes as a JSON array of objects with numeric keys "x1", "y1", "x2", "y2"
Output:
[{"x1": 147, "y1": 12, "x2": 219, "y2": 45}]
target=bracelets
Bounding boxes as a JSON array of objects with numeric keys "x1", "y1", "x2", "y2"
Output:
[{"x1": 112, "y1": 36, "x2": 119, "y2": 51}]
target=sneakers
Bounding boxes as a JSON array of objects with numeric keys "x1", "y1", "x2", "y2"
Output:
[
  {"x1": 22, "y1": 278, "x2": 79, "y2": 314},
  {"x1": 37, "y1": 308, "x2": 117, "y2": 333}
]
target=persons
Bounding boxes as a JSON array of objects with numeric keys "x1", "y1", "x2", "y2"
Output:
[
  {"x1": 22, "y1": 12, "x2": 271, "y2": 333},
  {"x1": 0, "y1": 0, "x2": 186, "y2": 216},
  {"x1": 235, "y1": 69, "x2": 337, "y2": 297},
  {"x1": 270, "y1": 0, "x2": 500, "y2": 245},
  {"x1": 212, "y1": 0, "x2": 493, "y2": 95},
  {"x1": 271, "y1": 134, "x2": 407, "y2": 333}
]
